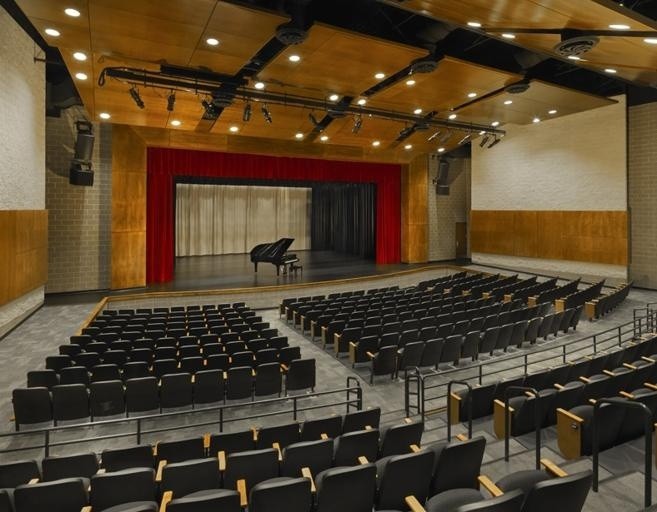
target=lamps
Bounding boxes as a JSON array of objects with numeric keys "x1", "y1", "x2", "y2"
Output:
[
  {"x1": 414, "y1": 109, "x2": 422, "y2": 115},
  {"x1": 320, "y1": 135, "x2": 329, "y2": 140},
  {"x1": 295, "y1": 133, "x2": 303, "y2": 139},
  {"x1": 492, "y1": 121, "x2": 500, "y2": 127},
  {"x1": 372, "y1": 141, "x2": 380, "y2": 147},
  {"x1": 229, "y1": 127, "x2": 238, "y2": 132},
  {"x1": 329, "y1": 95, "x2": 338, "y2": 102},
  {"x1": 468, "y1": 92, "x2": 476, "y2": 97},
  {"x1": 358, "y1": 99, "x2": 366, "y2": 105},
  {"x1": 479, "y1": 131, "x2": 486, "y2": 136},
  {"x1": 449, "y1": 114, "x2": 457, "y2": 120},
  {"x1": 405, "y1": 145, "x2": 412, "y2": 150},
  {"x1": 171, "y1": 120, "x2": 181, "y2": 127}
]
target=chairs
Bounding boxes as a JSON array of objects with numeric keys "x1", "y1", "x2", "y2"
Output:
[
  {"x1": 279, "y1": 272, "x2": 634, "y2": 386},
  {"x1": 448, "y1": 333, "x2": 657, "y2": 511},
  {"x1": 0, "y1": 407, "x2": 594, "y2": 512},
  {"x1": 13, "y1": 301, "x2": 316, "y2": 432}
]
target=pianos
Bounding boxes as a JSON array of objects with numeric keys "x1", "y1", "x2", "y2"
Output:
[
  {"x1": 283, "y1": 258, "x2": 299, "y2": 264},
  {"x1": 250, "y1": 238, "x2": 299, "y2": 275}
]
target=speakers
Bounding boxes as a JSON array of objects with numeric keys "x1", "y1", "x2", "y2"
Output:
[
  {"x1": 436, "y1": 185, "x2": 449, "y2": 195},
  {"x1": 75, "y1": 134, "x2": 94, "y2": 161},
  {"x1": 438, "y1": 162, "x2": 449, "y2": 185},
  {"x1": 69, "y1": 168, "x2": 94, "y2": 186}
]
